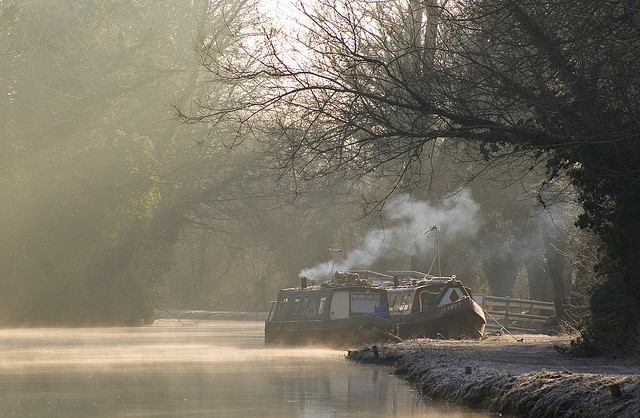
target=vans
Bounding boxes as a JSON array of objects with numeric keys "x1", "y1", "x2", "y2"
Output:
[{"x1": 431, "y1": 277, "x2": 451, "y2": 283}]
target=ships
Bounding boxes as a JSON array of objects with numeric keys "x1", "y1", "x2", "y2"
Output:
[
  {"x1": 264, "y1": 287, "x2": 392, "y2": 345},
  {"x1": 340, "y1": 277, "x2": 486, "y2": 340}
]
